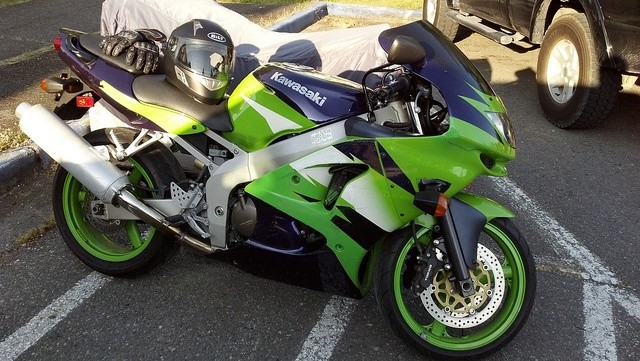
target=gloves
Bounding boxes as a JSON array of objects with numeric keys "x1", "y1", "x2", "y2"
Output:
[
  {"x1": 99, "y1": 28, "x2": 167, "y2": 57},
  {"x1": 126, "y1": 41, "x2": 159, "y2": 74}
]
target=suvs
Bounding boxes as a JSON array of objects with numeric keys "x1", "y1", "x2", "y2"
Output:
[{"x1": 423, "y1": 0, "x2": 640, "y2": 129}]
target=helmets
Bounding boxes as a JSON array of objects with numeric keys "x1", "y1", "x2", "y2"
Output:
[{"x1": 165, "y1": 19, "x2": 235, "y2": 105}]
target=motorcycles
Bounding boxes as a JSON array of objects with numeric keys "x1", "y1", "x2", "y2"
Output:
[{"x1": 14, "y1": 19, "x2": 536, "y2": 361}]
